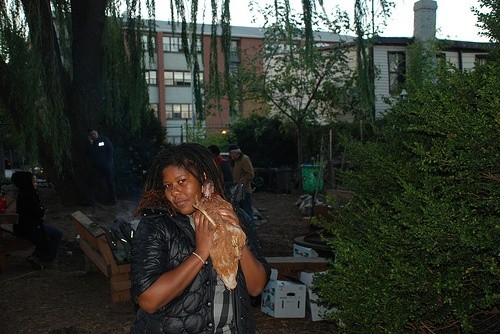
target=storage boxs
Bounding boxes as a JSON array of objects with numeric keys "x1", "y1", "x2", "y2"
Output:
[
  {"x1": 299, "y1": 271, "x2": 339, "y2": 322},
  {"x1": 293, "y1": 243, "x2": 319, "y2": 258},
  {"x1": 261, "y1": 269, "x2": 306, "y2": 319}
]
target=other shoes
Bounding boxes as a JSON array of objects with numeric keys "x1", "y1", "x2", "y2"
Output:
[{"x1": 26, "y1": 257, "x2": 47, "y2": 270}]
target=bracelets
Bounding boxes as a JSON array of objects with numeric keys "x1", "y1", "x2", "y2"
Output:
[{"x1": 192, "y1": 252, "x2": 205, "y2": 265}]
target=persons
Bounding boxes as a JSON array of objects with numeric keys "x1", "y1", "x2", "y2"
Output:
[
  {"x1": 11, "y1": 171, "x2": 67, "y2": 272},
  {"x1": 206, "y1": 144, "x2": 234, "y2": 194},
  {"x1": 0, "y1": 189, "x2": 7, "y2": 214},
  {"x1": 128, "y1": 143, "x2": 272, "y2": 334},
  {"x1": 79, "y1": 128, "x2": 119, "y2": 205},
  {"x1": 227, "y1": 143, "x2": 256, "y2": 231}
]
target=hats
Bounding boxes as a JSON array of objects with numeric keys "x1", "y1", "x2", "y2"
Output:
[{"x1": 229, "y1": 145, "x2": 240, "y2": 151}]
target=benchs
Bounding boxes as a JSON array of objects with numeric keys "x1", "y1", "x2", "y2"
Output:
[
  {"x1": 71, "y1": 209, "x2": 132, "y2": 303},
  {"x1": 1, "y1": 222, "x2": 37, "y2": 267}
]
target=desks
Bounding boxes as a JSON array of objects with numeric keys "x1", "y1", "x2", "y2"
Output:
[
  {"x1": 264, "y1": 255, "x2": 332, "y2": 280},
  {"x1": 0, "y1": 212, "x2": 19, "y2": 272}
]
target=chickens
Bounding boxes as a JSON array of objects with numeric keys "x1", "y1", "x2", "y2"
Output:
[{"x1": 192, "y1": 178, "x2": 248, "y2": 291}]
